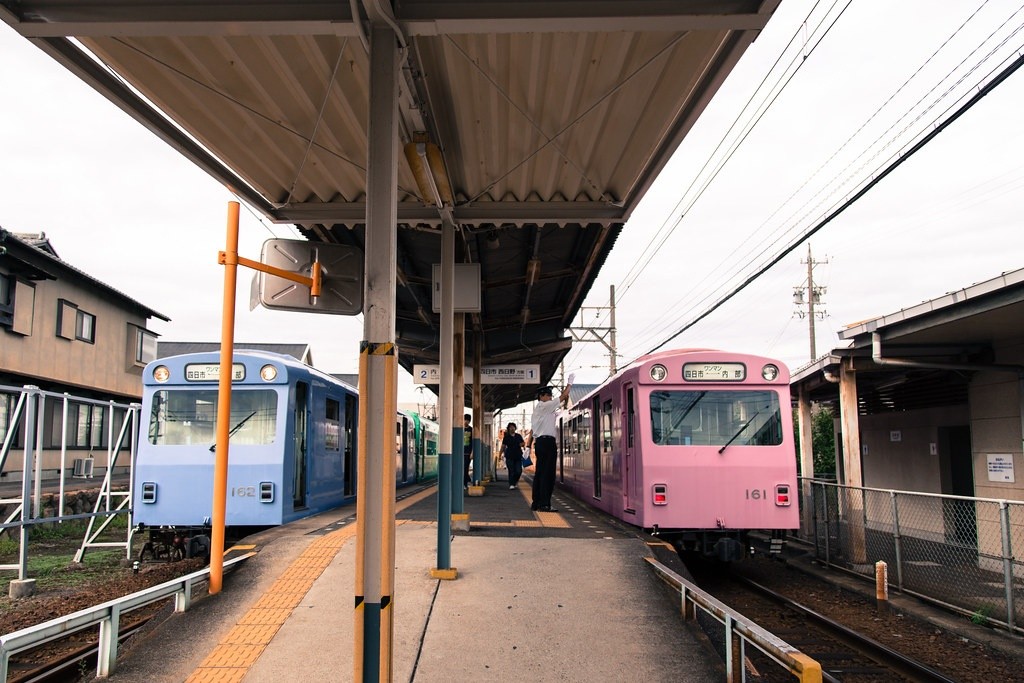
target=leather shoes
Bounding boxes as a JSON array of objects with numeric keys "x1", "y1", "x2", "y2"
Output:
[{"x1": 531, "y1": 505, "x2": 558, "y2": 512}]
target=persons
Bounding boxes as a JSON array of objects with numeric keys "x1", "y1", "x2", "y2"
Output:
[
  {"x1": 464, "y1": 414, "x2": 472, "y2": 491},
  {"x1": 523, "y1": 373, "x2": 575, "y2": 512},
  {"x1": 498, "y1": 422, "x2": 524, "y2": 490}
]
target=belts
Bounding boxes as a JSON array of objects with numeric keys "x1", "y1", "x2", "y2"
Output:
[{"x1": 535, "y1": 436, "x2": 555, "y2": 440}]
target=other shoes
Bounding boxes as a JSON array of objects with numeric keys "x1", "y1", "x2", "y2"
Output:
[{"x1": 509, "y1": 485, "x2": 517, "y2": 489}]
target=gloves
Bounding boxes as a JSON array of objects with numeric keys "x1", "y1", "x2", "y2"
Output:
[
  {"x1": 522, "y1": 448, "x2": 530, "y2": 460},
  {"x1": 568, "y1": 373, "x2": 577, "y2": 386}
]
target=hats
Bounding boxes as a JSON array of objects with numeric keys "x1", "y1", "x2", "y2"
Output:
[{"x1": 537, "y1": 386, "x2": 553, "y2": 394}]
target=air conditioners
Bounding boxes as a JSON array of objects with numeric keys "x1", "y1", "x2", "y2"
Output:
[{"x1": 72, "y1": 458, "x2": 95, "y2": 480}]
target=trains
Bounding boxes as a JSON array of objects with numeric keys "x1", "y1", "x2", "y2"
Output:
[
  {"x1": 554, "y1": 349, "x2": 800, "y2": 575},
  {"x1": 130, "y1": 348, "x2": 440, "y2": 577}
]
